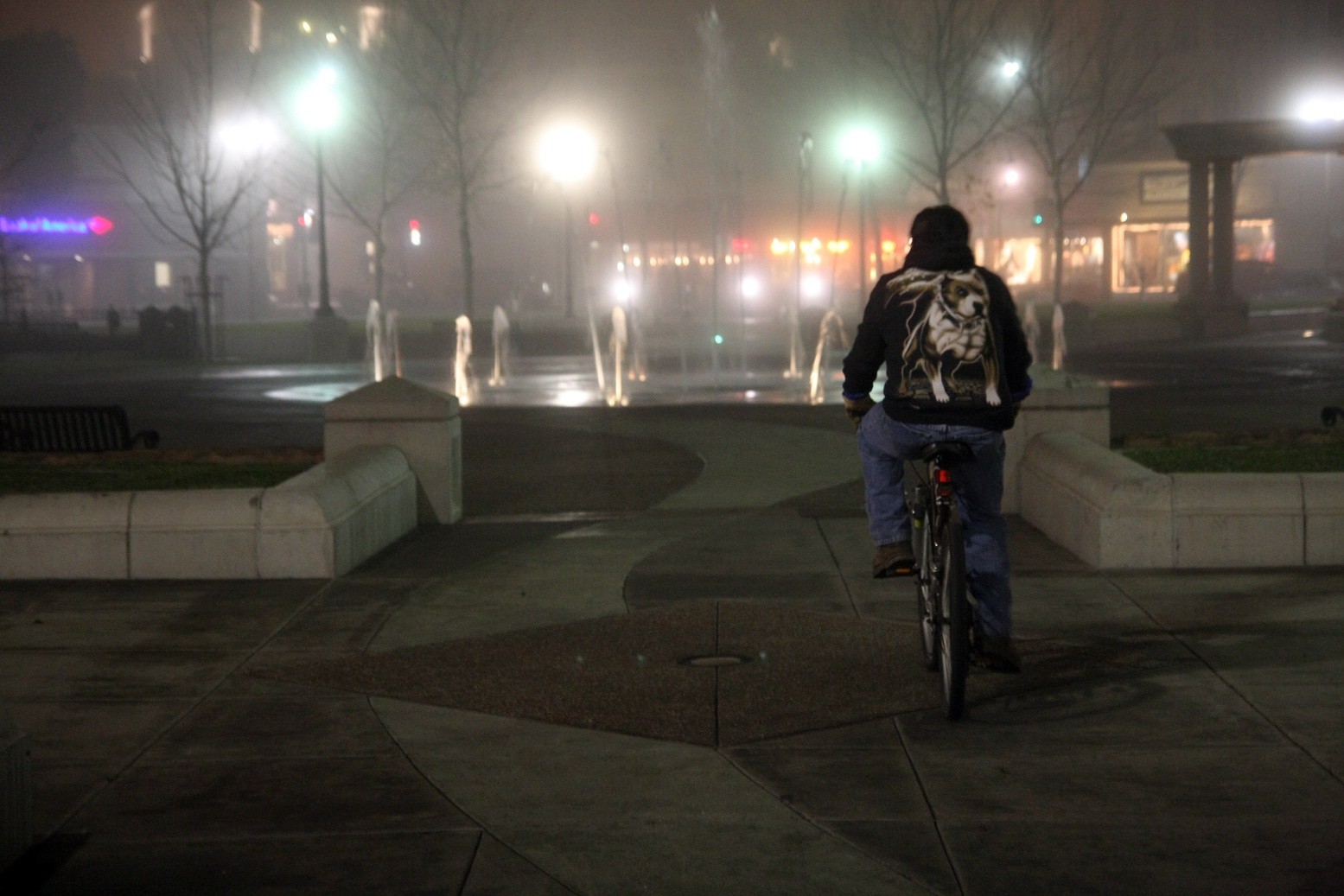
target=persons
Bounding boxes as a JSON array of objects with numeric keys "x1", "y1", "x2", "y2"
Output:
[
  {"x1": 841, "y1": 204, "x2": 1031, "y2": 668},
  {"x1": 106, "y1": 304, "x2": 119, "y2": 334}
]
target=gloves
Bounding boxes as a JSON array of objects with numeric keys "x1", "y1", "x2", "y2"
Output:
[
  {"x1": 842, "y1": 393, "x2": 877, "y2": 425},
  {"x1": 1006, "y1": 402, "x2": 1020, "y2": 430}
]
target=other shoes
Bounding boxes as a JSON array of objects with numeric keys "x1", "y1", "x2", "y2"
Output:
[
  {"x1": 973, "y1": 626, "x2": 1006, "y2": 666},
  {"x1": 873, "y1": 541, "x2": 915, "y2": 579}
]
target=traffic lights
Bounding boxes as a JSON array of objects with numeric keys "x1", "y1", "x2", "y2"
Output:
[{"x1": 1031, "y1": 196, "x2": 1049, "y2": 231}]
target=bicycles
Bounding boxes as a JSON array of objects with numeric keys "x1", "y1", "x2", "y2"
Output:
[{"x1": 894, "y1": 440, "x2": 996, "y2": 721}]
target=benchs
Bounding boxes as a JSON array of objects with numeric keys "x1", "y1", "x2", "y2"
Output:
[{"x1": 0, "y1": 402, "x2": 161, "y2": 458}]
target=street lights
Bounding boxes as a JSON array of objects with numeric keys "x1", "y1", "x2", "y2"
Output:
[
  {"x1": 287, "y1": 70, "x2": 364, "y2": 356},
  {"x1": 830, "y1": 117, "x2": 886, "y2": 313},
  {"x1": 542, "y1": 117, "x2": 600, "y2": 322}
]
